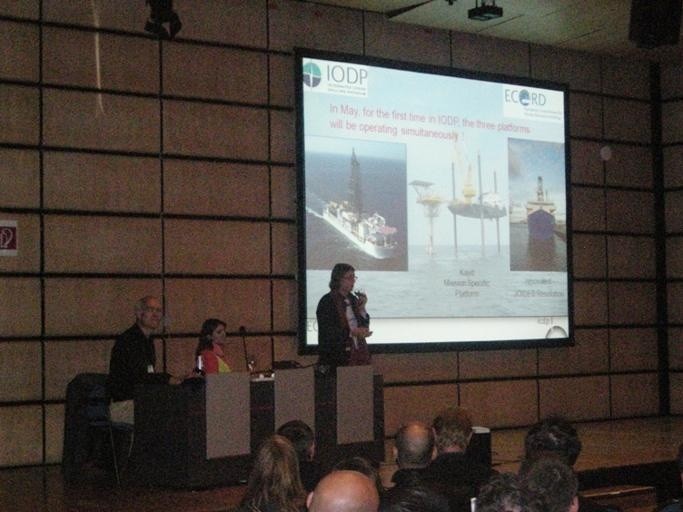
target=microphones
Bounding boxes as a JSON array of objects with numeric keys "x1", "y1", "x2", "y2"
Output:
[{"x1": 239, "y1": 325, "x2": 249, "y2": 373}]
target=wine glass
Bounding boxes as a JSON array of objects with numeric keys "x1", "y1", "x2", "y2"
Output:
[{"x1": 248, "y1": 355, "x2": 258, "y2": 377}]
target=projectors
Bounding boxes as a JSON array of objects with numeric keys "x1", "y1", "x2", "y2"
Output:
[{"x1": 468, "y1": 5, "x2": 503, "y2": 22}]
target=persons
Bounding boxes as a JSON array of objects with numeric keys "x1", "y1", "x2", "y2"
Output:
[
  {"x1": 194, "y1": 318, "x2": 233, "y2": 374},
  {"x1": 110, "y1": 295, "x2": 183, "y2": 385},
  {"x1": 659, "y1": 446, "x2": 683, "y2": 512},
  {"x1": 234, "y1": 406, "x2": 606, "y2": 512},
  {"x1": 316, "y1": 263, "x2": 373, "y2": 366}
]
